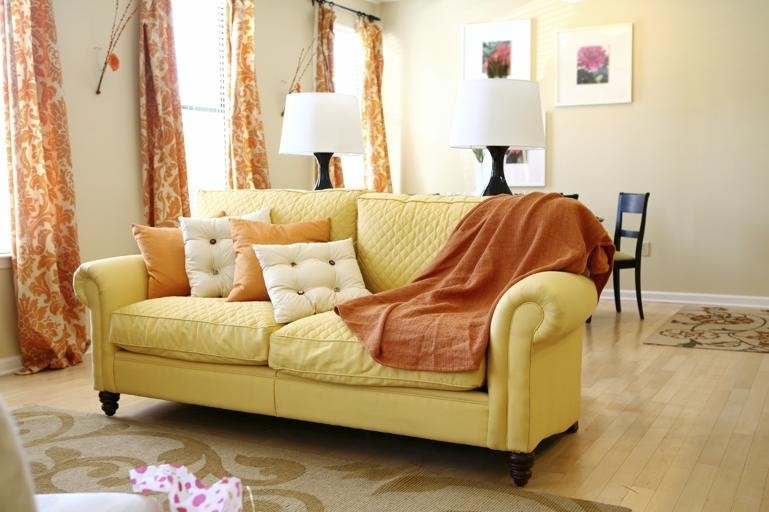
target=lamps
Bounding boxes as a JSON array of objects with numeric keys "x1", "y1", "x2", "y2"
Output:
[
  {"x1": 277, "y1": 92, "x2": 365, "y2": 191},
  {"x1": 448, "y1": 77, "x2": 546, "y2": 196}
]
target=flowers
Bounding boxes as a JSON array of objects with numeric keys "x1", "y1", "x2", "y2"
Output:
[
  {"x1": 281, "y1": 36, "x2": 320, "y2": 116},
  {"x1": 94, "y1": 0, "x2": 148, "y2": 95}
]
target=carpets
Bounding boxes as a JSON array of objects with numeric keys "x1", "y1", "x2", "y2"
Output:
[
  {"x1": 642, "y1": 304, "x2": 769, "y2": 353},
  {"x1": 6, "y1": 397, "x2": 634, "y2": 511}
]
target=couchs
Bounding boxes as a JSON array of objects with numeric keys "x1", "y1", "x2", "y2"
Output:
[{"x1": 72, "y1": 189, "x2": 598, "y2": 490}]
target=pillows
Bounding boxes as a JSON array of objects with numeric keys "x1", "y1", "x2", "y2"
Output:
[{"x1": 129, "y1": 206, "x2": 375, "y2": 328}]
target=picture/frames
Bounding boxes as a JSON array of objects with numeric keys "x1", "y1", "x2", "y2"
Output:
[
  {"x1": 472, "y1": 109, "x2": 545, "y2": 188},
  {"x1": 554, "y1": 22, "x2": 633, "y2": 107},
  {"x1": 458, "y1": 18, "x2": 531, "y2": 83}
]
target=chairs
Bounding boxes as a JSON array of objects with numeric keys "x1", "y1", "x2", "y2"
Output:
[{"x1": 585, "y1": 192, "x2": 650, "y2": 323}]
